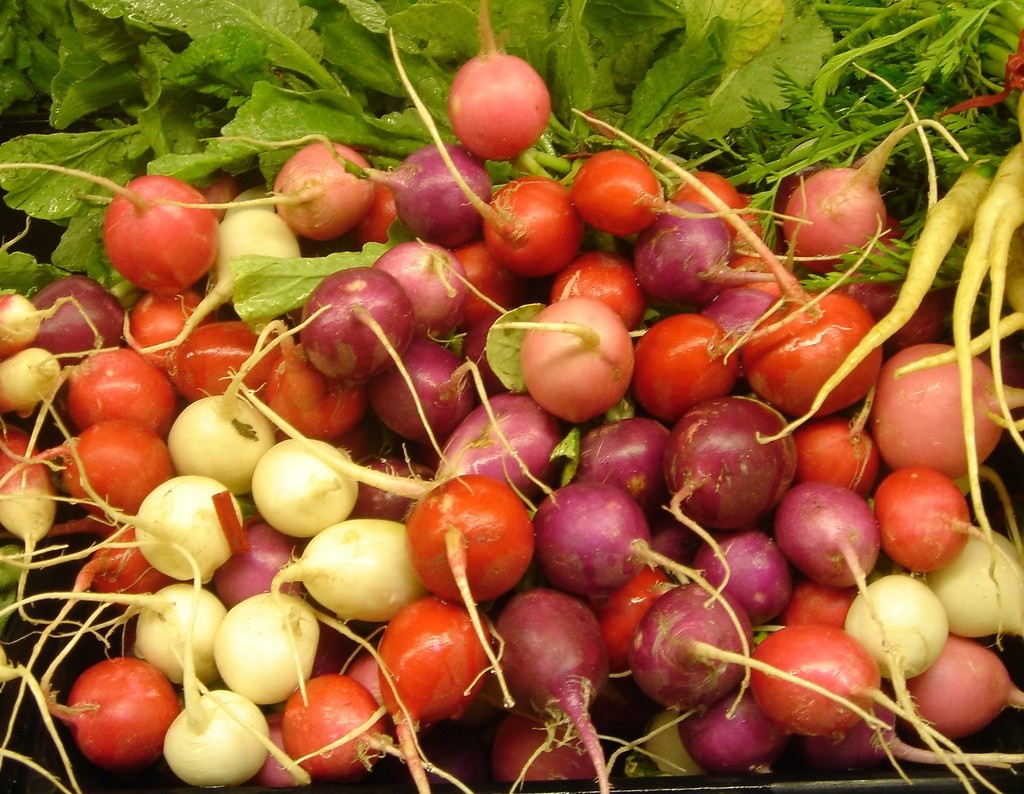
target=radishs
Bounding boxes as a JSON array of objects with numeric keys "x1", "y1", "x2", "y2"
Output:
[
  {"x1": 842, "y1": 521, "x2": 1024, "y2": 794},
  {"x1": 50, "y1": 319, "x2": 432, "y2": 788},
  {"x1": 124, "y1": 188, "x2": 302, "y2": 354}
]
